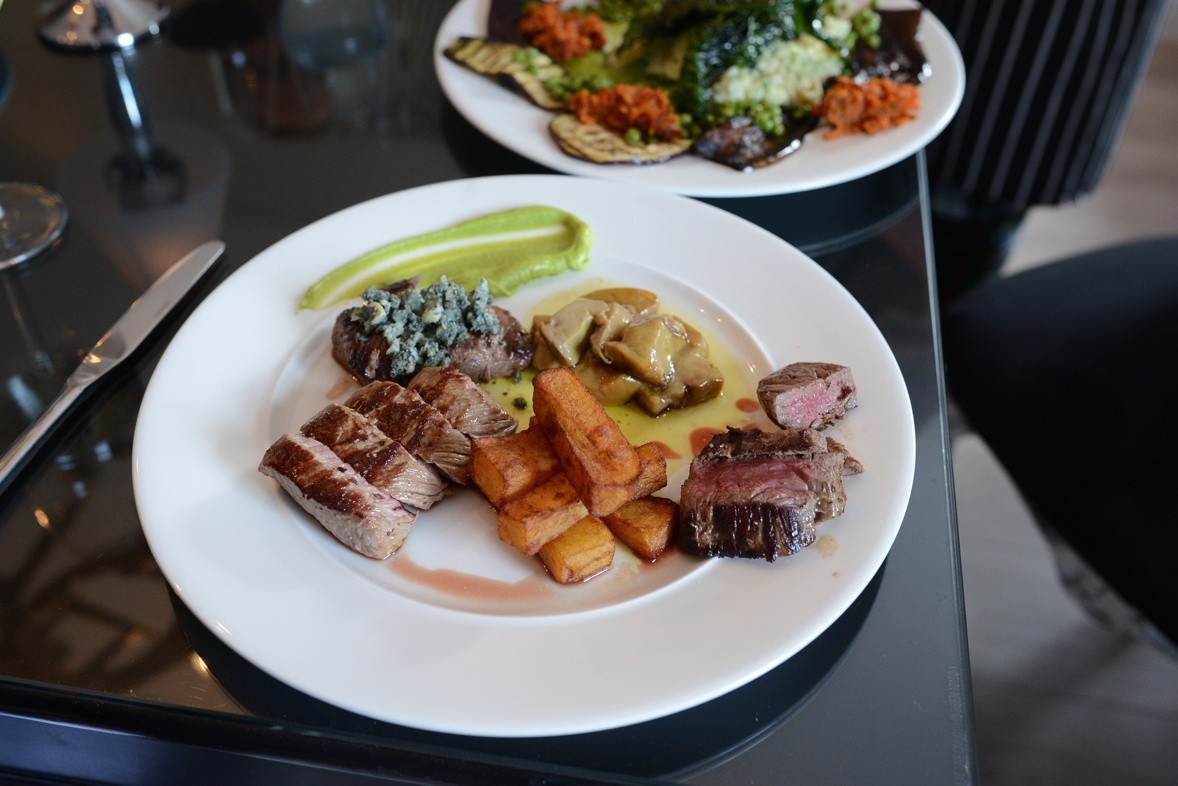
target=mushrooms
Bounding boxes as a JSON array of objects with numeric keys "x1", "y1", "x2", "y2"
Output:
[{"x1": 529, "y1": 298, "x2": 725, "y2": 413}]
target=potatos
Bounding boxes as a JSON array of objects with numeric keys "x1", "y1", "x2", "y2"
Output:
[{"x1": 471, "y1": 367, "x2": 679, "y2": 583}]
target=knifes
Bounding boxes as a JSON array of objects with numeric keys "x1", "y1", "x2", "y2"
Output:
[{"x1": 2, "y1": 240, "x2": 220, "y2": 492}]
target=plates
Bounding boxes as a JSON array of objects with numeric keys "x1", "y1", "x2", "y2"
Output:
[
  {"x1": 130, "y1": 172, "x2": 919, "y2": 743},
  {"x1": 431, "y1": 0, "x2": 964, "y2": 198}
]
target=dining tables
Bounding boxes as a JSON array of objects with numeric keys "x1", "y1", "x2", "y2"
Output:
[{"x1": 0, "y1": 0, "x2": 981, "y2": 785}]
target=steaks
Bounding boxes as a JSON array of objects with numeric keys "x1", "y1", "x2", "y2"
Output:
[
  {"x1": 258, "y1": 272, "x2": 533, "y2": 558},
  {"x1": 672, "y1": 360, "x2": 863, "y2": 557}
]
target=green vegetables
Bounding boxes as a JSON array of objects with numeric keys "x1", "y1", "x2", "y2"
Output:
[{"x1": 448, "y1": 0, "x2": 930, "y2": 168}]
